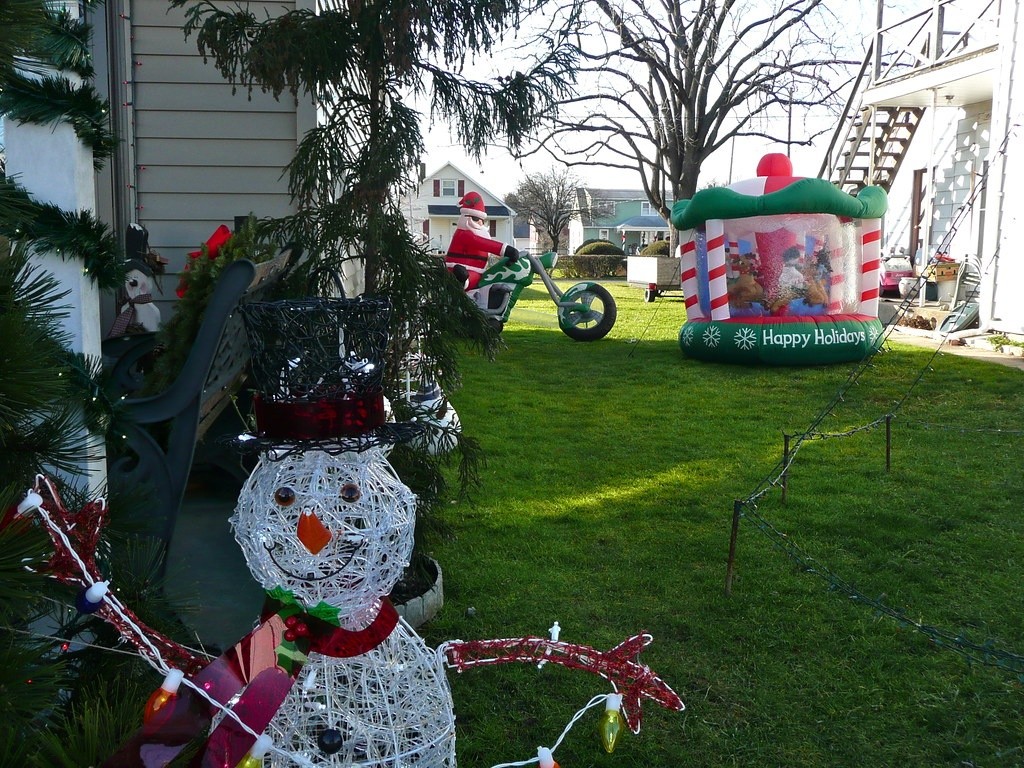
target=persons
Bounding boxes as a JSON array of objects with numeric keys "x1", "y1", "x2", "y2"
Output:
[{"x1": 446, "y1": 192, "x2": 519, "y2": 291}]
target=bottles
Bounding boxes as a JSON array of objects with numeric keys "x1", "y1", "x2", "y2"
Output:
[{"x1": 898, "y1": 276, "x2": 919, "y2": 299}]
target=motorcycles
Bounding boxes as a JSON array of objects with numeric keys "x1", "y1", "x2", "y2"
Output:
[{"x1": 422, "y1": 249, "x2": 618, "y2": 343}]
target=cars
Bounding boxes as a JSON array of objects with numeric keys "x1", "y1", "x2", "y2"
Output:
[{"x1": 879, "y1": 255, "x2": 913, "y2": 296}]
